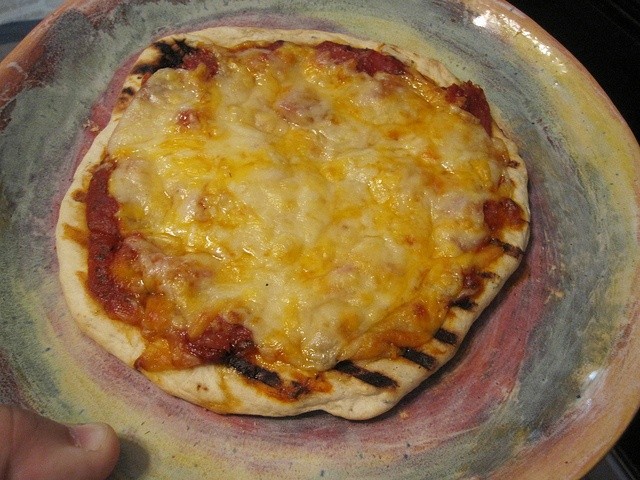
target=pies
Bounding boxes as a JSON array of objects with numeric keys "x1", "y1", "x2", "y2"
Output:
[{"x1": 55, "y1": 26, "x2": 531, "y2": 422}]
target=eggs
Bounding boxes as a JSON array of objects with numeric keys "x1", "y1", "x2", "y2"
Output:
[{"x1": 89, "y1": 67, "x2": 493, "y2": 390}]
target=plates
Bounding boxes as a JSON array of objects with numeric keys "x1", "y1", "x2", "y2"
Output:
[{"x1": 1, "y1": 0, "x2": 636, "y2": 478}]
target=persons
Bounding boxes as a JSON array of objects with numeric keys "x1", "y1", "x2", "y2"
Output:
[{"x1": 0, "y1": 395, "x2": 120, "y2": 479}]
